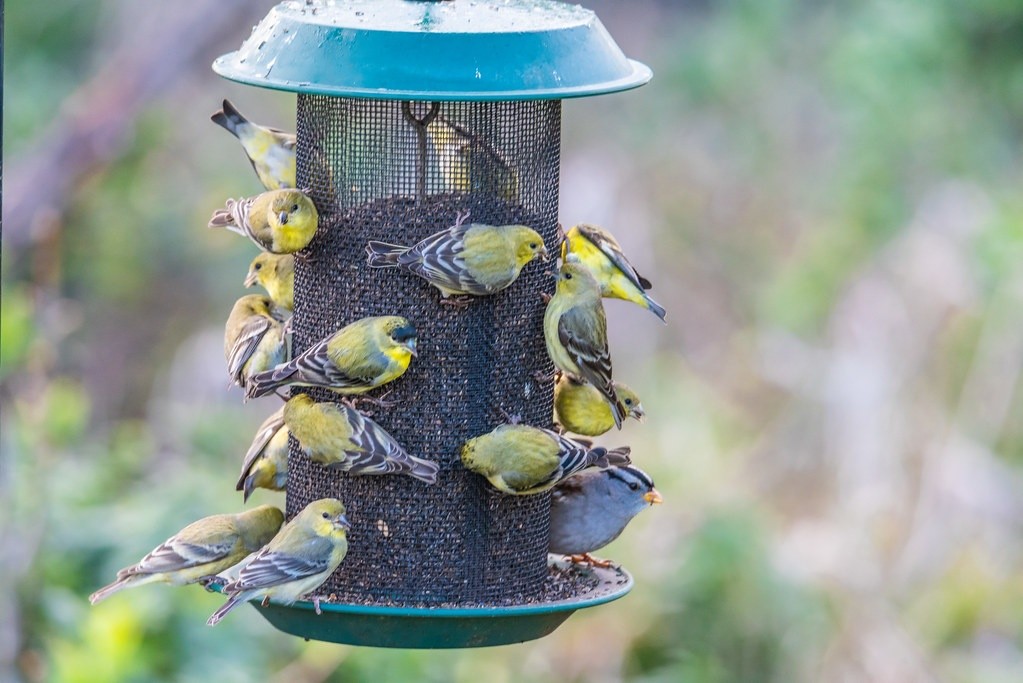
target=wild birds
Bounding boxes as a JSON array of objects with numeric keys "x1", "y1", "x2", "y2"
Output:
[{"x1": 88, "y1": 98, "x2": 668, "y2": 629}]
